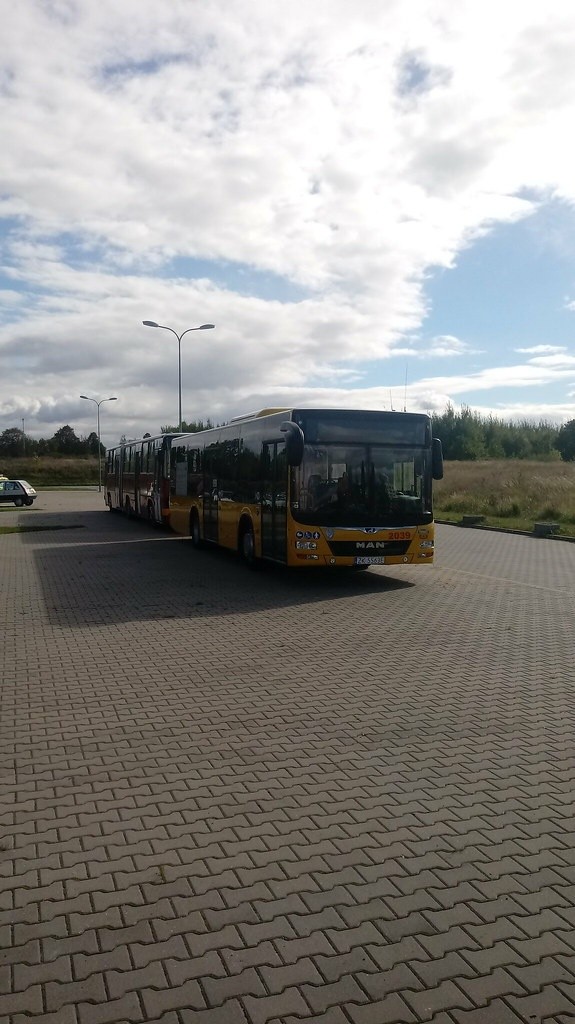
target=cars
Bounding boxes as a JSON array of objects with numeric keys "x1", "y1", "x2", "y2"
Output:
[{"x1": 0, "y1": 479, "x2": 37, "y2": 507}]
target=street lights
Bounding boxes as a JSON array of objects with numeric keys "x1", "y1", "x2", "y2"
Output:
[
  {"x1": 79, "y1": 395, "x2": 118, "y2": 492},
  {"x1": 143, "y1": 321, "x2": 215, "y2": 433}
]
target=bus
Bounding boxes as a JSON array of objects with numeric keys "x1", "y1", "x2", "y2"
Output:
[
  {"x1": 173, "y1": 408, "x2": 445, "y2": 574},
  {"x1": 103, "y1": 432, "x2": 209, "y2": 529}
]
